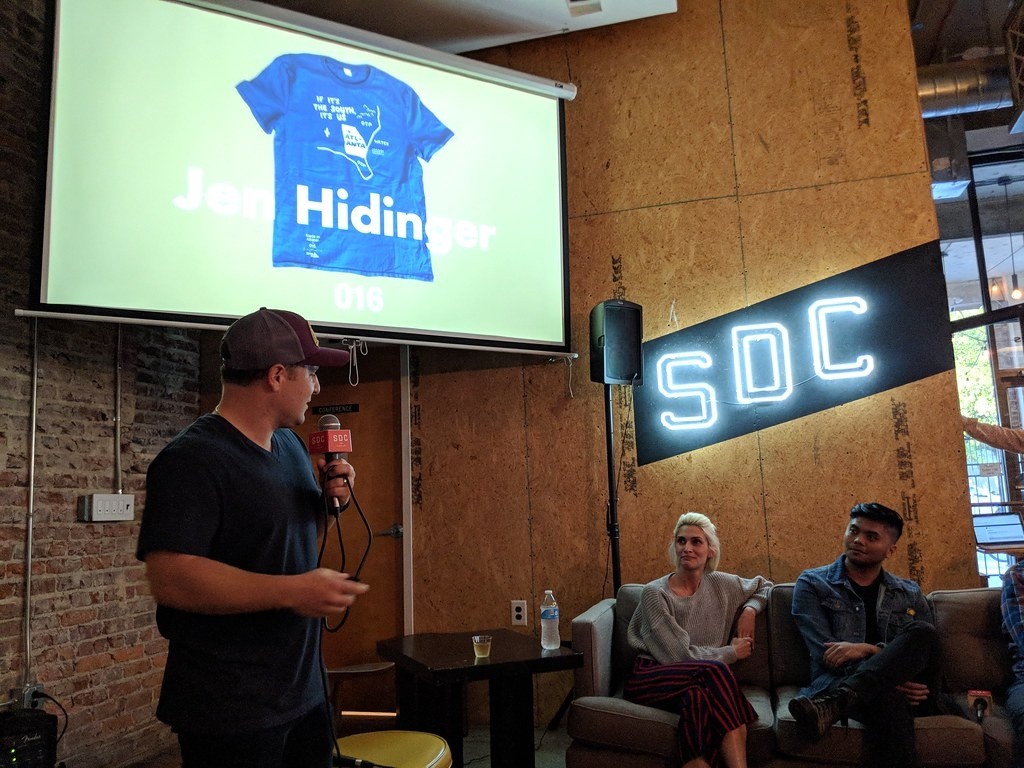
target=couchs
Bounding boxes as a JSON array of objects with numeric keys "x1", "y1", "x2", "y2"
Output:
[{"x1": 561, "y1": 582, "x2": 1024, "y2": 768}]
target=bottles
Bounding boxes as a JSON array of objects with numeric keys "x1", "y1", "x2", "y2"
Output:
[{"x1": 540, "y1": 589, "x2": 561, "y2": 650}]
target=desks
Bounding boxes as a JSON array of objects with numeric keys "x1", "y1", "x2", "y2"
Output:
[{"x1": 376, "y1": 626, "x2": 584, "y2": 768}]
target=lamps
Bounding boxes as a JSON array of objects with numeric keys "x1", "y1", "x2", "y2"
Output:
[{"x1": 998, "y1": 179, "x2": 1023, "y2": 300}]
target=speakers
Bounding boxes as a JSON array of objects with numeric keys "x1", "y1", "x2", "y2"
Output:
[
  {"x1": 589, "y1": 299, "x2": 645, "y2": 386},
  {"x1": 0, "y1": 709, "x2": 58, "y2": 768}
]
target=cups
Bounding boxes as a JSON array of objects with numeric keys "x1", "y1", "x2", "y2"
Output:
[{"x1": 472, "y1": 636, "x2": 492, "y2": 658}]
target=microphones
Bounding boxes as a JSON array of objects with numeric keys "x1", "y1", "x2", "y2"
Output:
[
  {"x1": 308, "y1": 414, "x2": 353, "y2": 516},
  {"x1": 967, "y1": 690, "x2": 993, "y2": 724}
]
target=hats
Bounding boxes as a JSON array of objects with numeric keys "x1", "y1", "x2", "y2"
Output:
[{"x1": 220, "y1": 307, "x2": 350, "y2": 371}]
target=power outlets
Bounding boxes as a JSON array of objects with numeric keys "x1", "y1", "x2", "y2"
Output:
[{"x1": 12, "y1": 684, "x2": 45, "y2": 710}]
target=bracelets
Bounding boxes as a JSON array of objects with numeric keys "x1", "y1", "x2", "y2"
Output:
[{"x1": 328, "y1": 498, "x2": 350, "y2": 515}]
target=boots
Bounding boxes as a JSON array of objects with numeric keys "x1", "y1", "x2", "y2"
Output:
[{"x1": 788, "y1": 687, "x2": 860, "y2": 743}]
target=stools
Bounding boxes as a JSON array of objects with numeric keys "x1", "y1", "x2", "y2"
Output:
[{"x1": 333, "y1": 730, "x2": 454, "y2": 768}]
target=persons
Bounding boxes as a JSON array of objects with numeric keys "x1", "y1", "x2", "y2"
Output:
[
  {"x1": 627, "y1": 512, "x2": 774, "y2": 768},
  {"x1": 136, "y1": 308, "x2": 372, "y2": 768},
  {"x1": 1001, "y1": 560, "x2": 1024, "y2": 742},
  {"x1": 792, "y1": 502, "x2": 941, "y2": 768},
  {"x1": 962, "y1": 415, "x2": 1024, "y2": 455}
]
